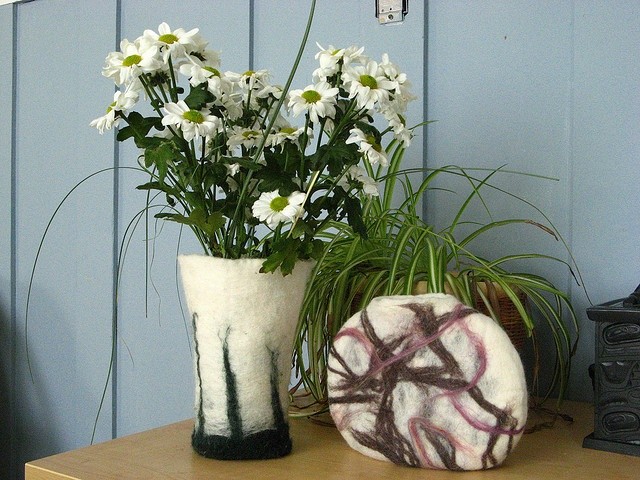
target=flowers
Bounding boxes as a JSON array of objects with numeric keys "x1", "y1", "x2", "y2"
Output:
[{"x1": 23, "y1": 0, "x2": 415, "y2": 429}]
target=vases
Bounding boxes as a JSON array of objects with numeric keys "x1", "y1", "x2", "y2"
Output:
[{"x1": 176, "y1": 253, "x2": 317, "y2": 460}]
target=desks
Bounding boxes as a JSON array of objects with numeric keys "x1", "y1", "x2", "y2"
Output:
[{"x1": 23, "y1": 394, "x2": 640, "y2": 480}]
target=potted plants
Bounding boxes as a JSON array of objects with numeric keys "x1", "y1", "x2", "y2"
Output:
[{"x1": 285, "y1": 115, "x2": 591, "y2": 433}]
